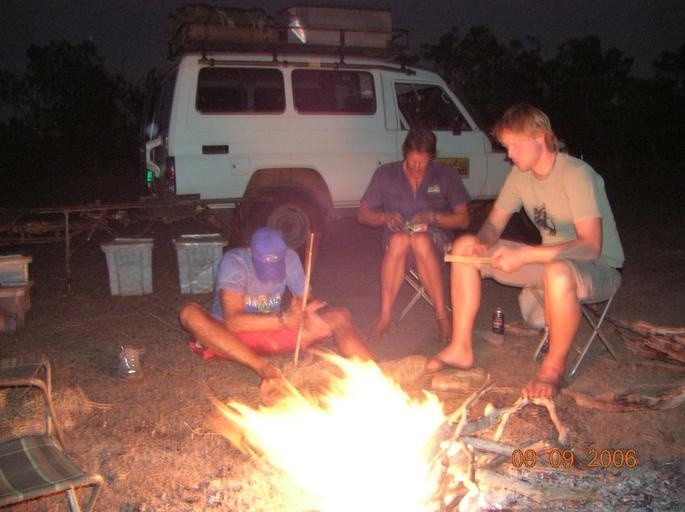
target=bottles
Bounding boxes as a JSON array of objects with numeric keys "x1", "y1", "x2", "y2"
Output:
[
  {"x1": 492, "y1": 305, "x2": 504, "y2": 333},
  {"x1": 118, "y1": 348, "x2": 141, "y2": 381}
]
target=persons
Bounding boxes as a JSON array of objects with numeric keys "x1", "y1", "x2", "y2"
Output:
[
  {"x1": 356, "y1": 128, "x2": 471, "y2": 343},
  {"x1": 425, "y1": 105, "x2": 626, "y2": 400},
  {"x1": 177, "y1": 227, "x2": 427, "y2": 408}
]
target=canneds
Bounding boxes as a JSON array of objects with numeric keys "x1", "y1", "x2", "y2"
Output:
[
  {"x1": 492, "y1": 309, "x2": 504, "y2": 335},
  {"x1": 539, "y1": 327, "x2": 549, "y2": 354}
]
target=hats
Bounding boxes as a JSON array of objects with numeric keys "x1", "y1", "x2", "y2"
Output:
[{"x1": 250, "y1": 227, "x2": 288, "y2": 284}]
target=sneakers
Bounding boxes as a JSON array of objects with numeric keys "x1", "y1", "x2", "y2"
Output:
[{"x1": 116, "y1": 345, "x2": 145, "y2": 384}]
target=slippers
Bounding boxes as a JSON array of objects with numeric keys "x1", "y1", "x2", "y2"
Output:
[
  {"x1": 426, "y1": 353, "x2": 474, "y2": 374},
  {"x1": 525, "y1": 374, "x2": 563, "y2": 400}
]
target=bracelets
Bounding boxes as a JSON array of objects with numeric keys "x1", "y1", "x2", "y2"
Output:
[
  {"x1": 277, "y1": 311, "x2": 286, "y2": 330},
  {"x1": 430, "y1": 211, "x2": 441, "y2": 226}
]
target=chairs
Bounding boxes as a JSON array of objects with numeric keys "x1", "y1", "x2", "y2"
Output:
[
  {"x1": 0, "y1": 349, "x2": 68, "y2": 452},
  {"x1": 0, "y1": 433, "x2": 104, "y2": 512}
]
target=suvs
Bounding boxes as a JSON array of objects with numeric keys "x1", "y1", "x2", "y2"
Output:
[{"x1": 134, "y1": 7, "x2": 572, "y2": 262}]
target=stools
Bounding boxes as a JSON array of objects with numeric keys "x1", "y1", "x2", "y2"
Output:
[
  {"x1": 533, "y1": 288, "x2": 620, "y2": 377},
  {"x1": 397, "y1": 262, "x2": 453, "y2": 322}
]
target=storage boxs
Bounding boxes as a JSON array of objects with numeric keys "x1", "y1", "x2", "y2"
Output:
[
  {"x1": 99, "y1": 235, "x2": 154, "y2": 297},
  {"x1": 170, "y1": 234, "x2": 231, "y2": 295},
  {"x1": 0, "y1": 252, "x2": 37, "y2": 334}
]
